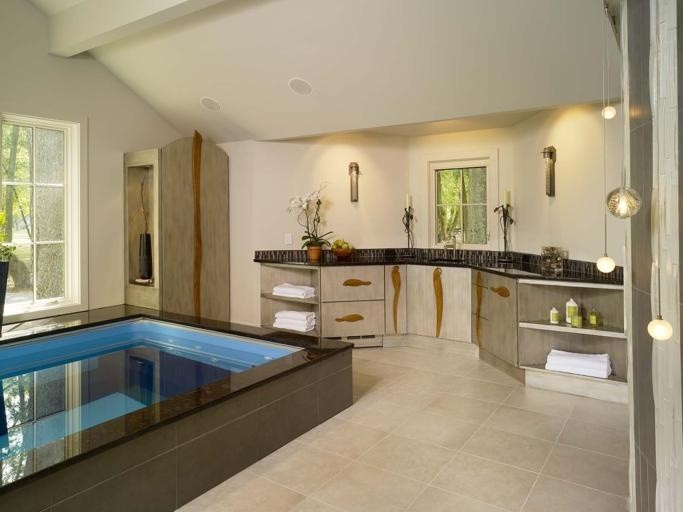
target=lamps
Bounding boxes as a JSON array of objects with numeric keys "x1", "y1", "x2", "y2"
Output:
[{"x1": 591, "y1": 1, "x2": 673, "y2": 342}]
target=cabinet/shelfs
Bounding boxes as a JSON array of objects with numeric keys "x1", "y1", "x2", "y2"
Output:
[
  {"x1": 259, "y1": 263, "x2": 471, "y2": 351},
  {"x1": 121, "y1": 129, "x2": 232, "y2": 336},
  {"x1": 472, "y1": 267, "x2": 631, "y2": 407}
]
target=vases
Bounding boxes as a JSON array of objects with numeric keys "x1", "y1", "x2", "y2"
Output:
[{"x1": 138, "y1": 231, "x2": 153, "y2": 280}]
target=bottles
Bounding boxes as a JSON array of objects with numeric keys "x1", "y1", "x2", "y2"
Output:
[
  {"x1": 550, "y1": 306, "x2": 559, "y2": 324},
  {"x1": 565, "y1": 299, "x2": 578, "y2": 324},
  {"x1": 590, "y1": 309, "x2": 598, "y2": 327}
]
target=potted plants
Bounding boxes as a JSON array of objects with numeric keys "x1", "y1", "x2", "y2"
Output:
[{"x1": 288, "y1": 180, "x2": 334, "y2": 265}]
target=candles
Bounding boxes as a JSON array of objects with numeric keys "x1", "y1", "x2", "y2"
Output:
[
  {"x1": 405, "y1": 192, "x2": 410, "y2": 210},
  {"x1": 503, "y1": 189, "x2": 509, "y2": 209}
]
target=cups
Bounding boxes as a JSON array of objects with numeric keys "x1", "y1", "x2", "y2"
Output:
[{"x1": 571, "y1": 312, "x2": 583, "y2": 327}]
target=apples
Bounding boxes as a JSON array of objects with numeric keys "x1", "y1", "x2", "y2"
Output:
[{"x1": 331, "y1": 239, "x2": 352, "y2": 250}]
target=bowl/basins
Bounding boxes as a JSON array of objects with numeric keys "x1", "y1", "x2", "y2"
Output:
[{"x1": 331, "y1": 248, "x2": 351, "y2": 258}]
